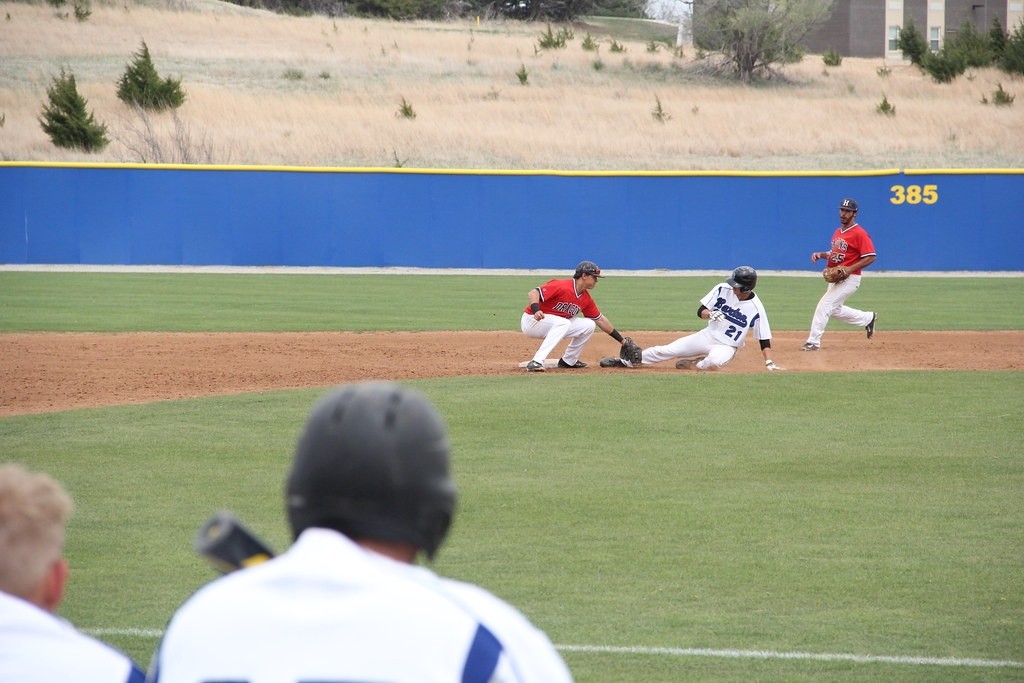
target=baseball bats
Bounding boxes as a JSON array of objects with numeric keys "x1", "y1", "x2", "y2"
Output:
[{"x1": 195, "y1": 515, "x2": 277, "y2": 574}]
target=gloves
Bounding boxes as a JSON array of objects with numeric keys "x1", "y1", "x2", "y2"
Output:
[
  {"x1": 765, "y1": 360, "x2": 786, "y2": 372},
  {"x1": 709, "y1": 311, "x2": 727, "y2": 322}
]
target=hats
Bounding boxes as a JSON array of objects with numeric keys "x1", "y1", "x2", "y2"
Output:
[
  {"x1": 838, "y1": 198, "x2": 862, "y2": 211},
  {"x1": 577, "y1": 261, "x2": 605, "y2": 279}
]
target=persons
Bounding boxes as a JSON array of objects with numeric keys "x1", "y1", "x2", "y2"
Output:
[
  {"x1": 799, "y1": 198, "x2": 877, "y2": 351},
  {"x1": 521, "y1": 261, "x2": 642, "y2": 372},
  {"x1": 600, "y1": 265, "x2": 786, "y2": 371},
  {"x1": 0, "y1": 464, "x2": 144, "y2": 683},
  {"x1": 145, "y1": 387, "x2": 575, "y2": 682}
]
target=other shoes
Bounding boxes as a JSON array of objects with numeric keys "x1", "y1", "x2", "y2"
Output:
[
  {"x1": 598, "y1": 356, "x2": 627, "y2": 368},
  {"x1": 801, "y1": 343, "x2": 820, "y2": 352},
  {"x1": 674, "y1": 355, "x2": 705, "y2": 371},
  {"x1": 865, "y1": 311, "x2": 878, "y2": 339}
]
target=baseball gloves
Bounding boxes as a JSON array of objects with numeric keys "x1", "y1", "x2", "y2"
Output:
[
  {"x1": 823, "y1": 265, "x2": 850, "y2": 286},
  {"x1": 620, "y1": 336, "x2": 642, "y2": 366}
]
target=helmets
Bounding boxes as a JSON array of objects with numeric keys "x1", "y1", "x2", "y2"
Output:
[
  {"x1": 282, "y1": 389, "x2": 455, "y2": 560},
  {"x1": 724, "y1": 264, "x2": 761, "y2": 290}
]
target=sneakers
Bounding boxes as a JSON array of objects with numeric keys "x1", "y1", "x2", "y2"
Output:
[
  {"x1": 557, "y1": 357, "x2": 589, "y2": 368},
  {"x1": 525, "y1": 360, "x2": 548, "y2": 373}
]
